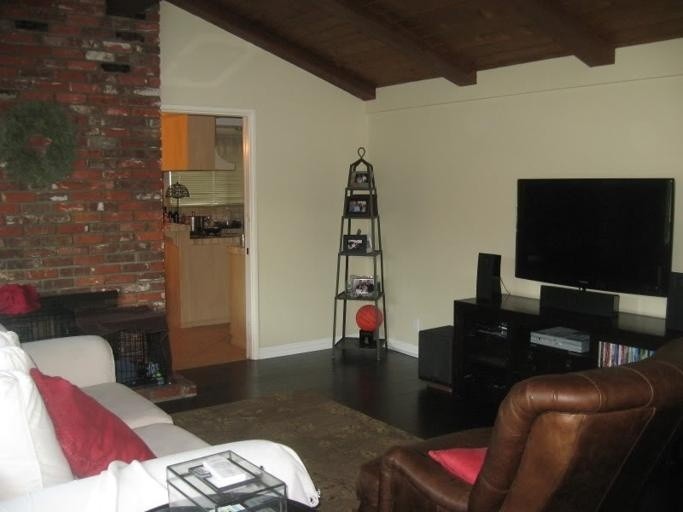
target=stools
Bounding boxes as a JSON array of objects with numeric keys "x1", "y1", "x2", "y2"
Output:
[{"x1": 417, "y1": 325, "x2": 455, "y2": 392}]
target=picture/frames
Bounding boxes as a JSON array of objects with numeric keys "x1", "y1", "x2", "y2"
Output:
[
  {"x1": 348, "y1": 274, "x2": 378, "y2": 299},
  {"x1": 351, "y1": 171, "x2": 372, "y2": 188},
  {"x1": 345, "y1": 196, "x2": 369, "y2": 218},
  {"x1": 343, "y1": 234, "x2": 367, "y2": 255}
]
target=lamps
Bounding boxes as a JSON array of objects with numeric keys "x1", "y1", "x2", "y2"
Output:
[{"x1": 166, "y1": 181, "x2": 189, "y2": 214}]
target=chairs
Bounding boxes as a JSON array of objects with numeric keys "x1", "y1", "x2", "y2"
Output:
[{"x1": 355, "y1": 337, "x2": 682, "y2": 511}]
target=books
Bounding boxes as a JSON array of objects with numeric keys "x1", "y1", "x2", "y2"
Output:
[{"x1": 598, "y1": 340, "x2": 654, "y2": 368}]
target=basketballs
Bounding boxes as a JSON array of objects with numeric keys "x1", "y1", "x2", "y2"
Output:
[{"x1": 356, "y1": 305, "x2": 383, "y2": 332}]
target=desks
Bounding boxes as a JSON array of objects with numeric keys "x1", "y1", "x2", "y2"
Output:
[{"x1": 146, "y1": 495, "x2": 319, "y2": 512}]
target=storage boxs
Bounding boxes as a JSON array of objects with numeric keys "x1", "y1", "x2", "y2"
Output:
[{"x1": 167, "y1": 451, "x2": 288, "y2": 512}]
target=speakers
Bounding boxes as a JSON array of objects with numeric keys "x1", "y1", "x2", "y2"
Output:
[
  {"x1": 665, "y1": 272, "x2": 682, "y2": 334},
  {"x1": 475, "y1": 252, "x2": 502, "y2": 305}
]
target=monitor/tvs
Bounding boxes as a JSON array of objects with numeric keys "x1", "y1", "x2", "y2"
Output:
[{"x1": 514, "y1": 178, "x2": 675, "y2": 298}]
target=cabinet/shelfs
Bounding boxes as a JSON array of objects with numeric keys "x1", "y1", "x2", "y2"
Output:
[
  {"x1": 166, "y1": 238, "x2": 238, "y2": 328},
  {"x1": 455, "y1": 293, "x2": 683, "y2": 410},
  {"x1": 331, "y1": 145, "x2": 390, "y2": 364},
  {"x1": 161, "y1": 112, "x2": 218, "y2": 171},
  {"x1": 227, "y1": 244, "x2": 246, "y2": 352}
]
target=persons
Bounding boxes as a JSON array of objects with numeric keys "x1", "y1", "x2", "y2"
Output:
[{"x1": 356, "y1": 281, "x2": 373, "y2": 291}]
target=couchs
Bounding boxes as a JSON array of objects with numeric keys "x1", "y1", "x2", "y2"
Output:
[{"x1": 1, "y1": 323, "x2": 320, "y2": 512}]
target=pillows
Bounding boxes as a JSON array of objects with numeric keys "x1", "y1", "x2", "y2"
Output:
[
  {"x1": 30, "y1": 369, "x2": 158, "y2": 481},
  {"x1": 426, "y1": 448, "x2": 490, "y2": 485}
]
